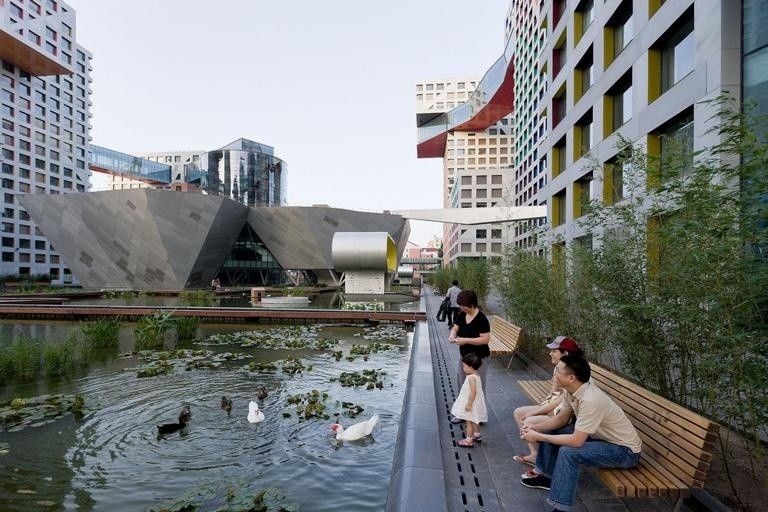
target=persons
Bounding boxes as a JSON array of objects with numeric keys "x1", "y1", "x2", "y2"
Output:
[
  {"x1": 519, "y1": 353, "x2": 642, "y2": 511},
  {"x1": 211, "y1": 278, "x2": 220, "y2": 291},
  {"x1": 512, "y1": 334, "x2": 594, "y2": 479},
  {"x1": 449, "y1": 352, "x2": 489, "y2": 448},
  {"x1": 448, "y1": 289, "x2": 492, "y2": 426},
  {"x1": 442, "y1": 278, "x2": 464, "y2": 330}
]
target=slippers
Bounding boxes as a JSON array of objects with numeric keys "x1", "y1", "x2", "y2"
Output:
[{"x1": 513, "y1": 454, "x2": 536, "y2": 467}]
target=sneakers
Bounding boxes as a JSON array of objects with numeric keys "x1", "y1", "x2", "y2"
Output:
[
  {"x1": 451, "y1": 416, "x2": 464, "y2": 424},
  {"x1": 521, "y1": 475, "x2": 554, "y2": 493}
]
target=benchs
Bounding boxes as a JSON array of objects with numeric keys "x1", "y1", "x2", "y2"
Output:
[
  {"x1": 487, "y1": 314, "x2": 524, "y2": 370},
  {"x1": 516, "y1": 362, "x2": 721, "y2": 511},
  {"x1": 251, "y1": 287, "x2": 320, "y2": 297}
]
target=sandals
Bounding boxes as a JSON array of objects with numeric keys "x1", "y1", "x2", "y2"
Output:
[
  {"x1": 473, "y1": 434, "x2": 483, "y2": 443},
  {"x1": 456, "y1": 438, "x2": 474, "y2": 448}
]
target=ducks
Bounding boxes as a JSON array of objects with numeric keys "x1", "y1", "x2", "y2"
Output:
[
  {"x1": 221, "y1": 395, "x2": 233, "y2": 410},
  {"x1": 328, "y1": 413, "x2": 380, "y2": 441},
  {"x1": 157, "y1": 409, "x2": 186, "y2": 435},
  {"x1": 257, "y1": 386, "x2": 268, "y2": 400},
  {"x1": 247, "y1": 400, "x2": 265, "y2": 424},
  {"x1": 180, "y1": 405, "x2": 191, "y2": 420}
]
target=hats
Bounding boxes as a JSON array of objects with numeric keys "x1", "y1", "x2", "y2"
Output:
[{"x1": 545, "y1": 336, "x2": 579, "y2": 353}]
